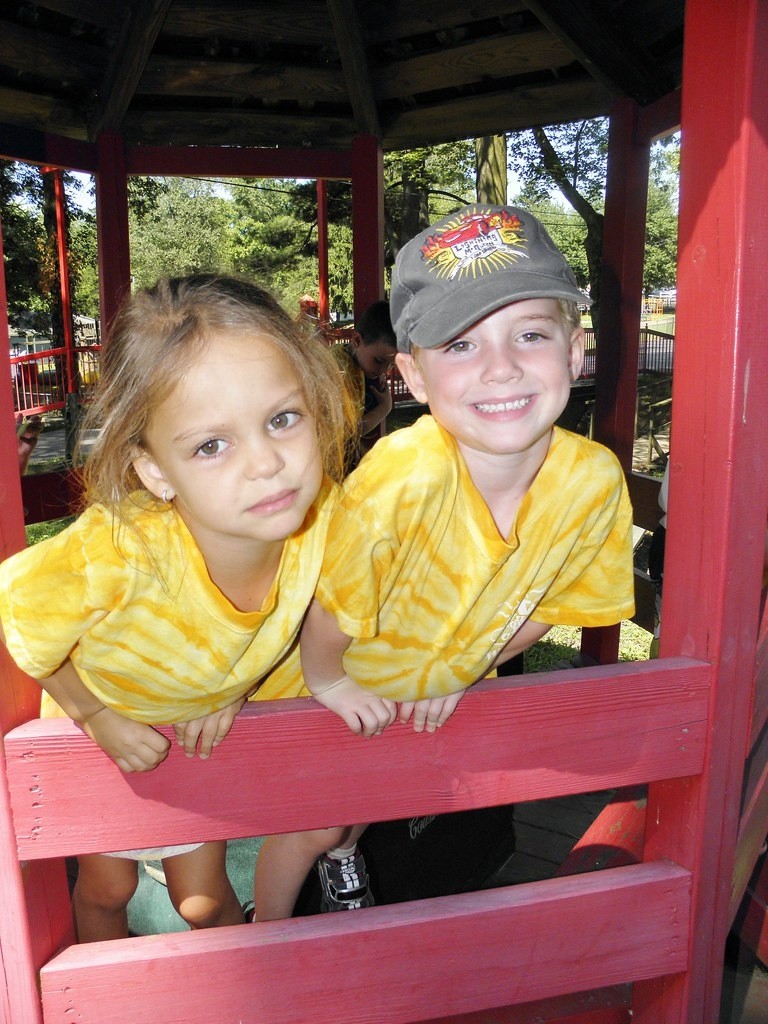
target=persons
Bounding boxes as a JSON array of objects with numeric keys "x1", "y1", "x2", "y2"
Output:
[
  {"x1": 329, "y1": 301, "x2": 398, "y2": 480},
  {"x1": 0, "y1": 274, "x2": 364, "y2": 944},
  {"x1": 300, "y1": 203, "x2": 635, "y2": 914}
]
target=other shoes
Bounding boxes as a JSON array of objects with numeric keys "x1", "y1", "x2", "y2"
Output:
[
  {"x1": 312, "y1": 849, "x2": 374, "y2": 911},
  {"x1": 242, "y1": 898, "x2": 258, "y2": 925}
]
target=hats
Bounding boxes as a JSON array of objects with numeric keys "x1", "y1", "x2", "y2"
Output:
[{"x1": 389, "y1": 202, "x2": 594, "y2": 352}]
